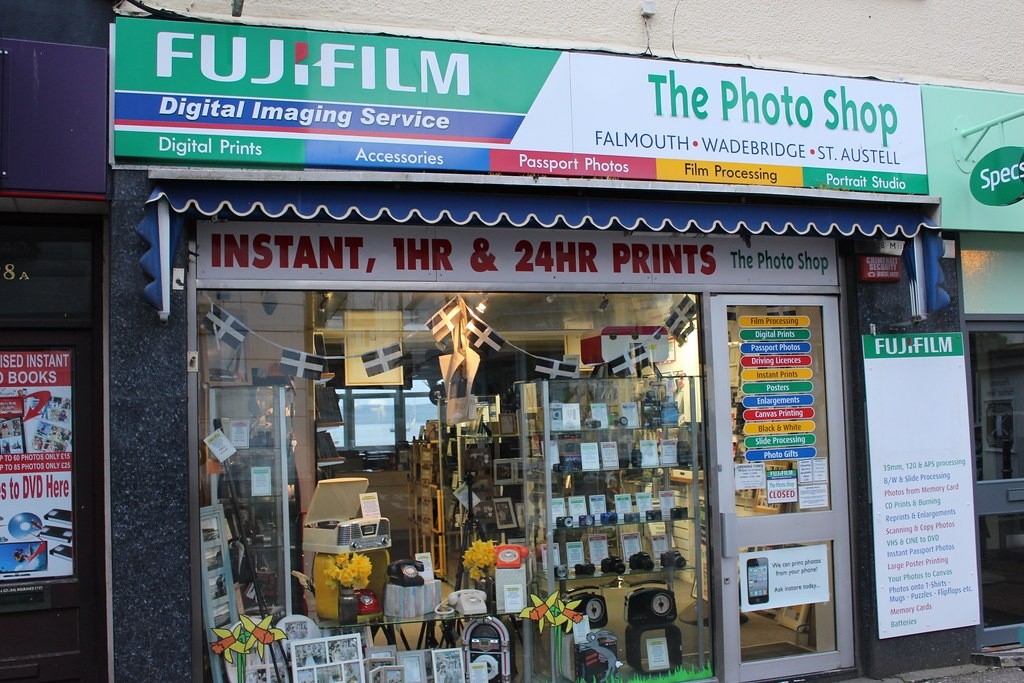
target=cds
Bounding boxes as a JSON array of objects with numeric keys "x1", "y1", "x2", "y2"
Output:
[{"x1": 7, "y1": 512, "x2": 43, "y2": 540}]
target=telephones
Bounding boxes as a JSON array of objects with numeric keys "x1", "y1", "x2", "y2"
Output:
[
  {"x1": 387, "y1": 557, "x2": 426, "y2": 588},
  {"x1": 490, "y1": 543, "x2": 529, "y2": 570},
  {"x1": 356, "y1": 588, "x2": 380, "y2": 615},
  {"x1": 445, "y1": 589, "x2": 489, "y2": 615}
]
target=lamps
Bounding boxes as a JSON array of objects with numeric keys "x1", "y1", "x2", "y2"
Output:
[
  {"x1": 546, "y1": 294, "x2": 559, "y2": 304},
  {"x1": 596, "y1": 294, "x2": 610, "y2": 312},
  {"x1": 317, "y1": 292, "x2": 329, "y2": 312},
  {"x1": 475, "y1": 294, "x2": 488, "y2": 313}
]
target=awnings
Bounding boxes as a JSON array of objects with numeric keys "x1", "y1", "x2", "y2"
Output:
[{"x1": 137, "y1": 182, "x2": 953, "y2": 323}]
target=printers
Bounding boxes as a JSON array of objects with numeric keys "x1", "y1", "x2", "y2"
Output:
[{"x1": 302, "y1": 478, "x2": 391, "y2": 554}]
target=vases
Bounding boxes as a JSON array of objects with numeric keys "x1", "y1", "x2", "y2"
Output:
[
  {"x1": 338, "y1": 589, "x2": 357, "y2": 625},
  {"x1": 475, "y1": 577, "x2": 493, "y2": 614}
]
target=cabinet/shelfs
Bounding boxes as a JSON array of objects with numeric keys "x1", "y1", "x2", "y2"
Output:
[
  {"x1": 304, "y1": 291, "x2": 346, "y2": 487},
  {"x1": 208, "y1": 383, "x2": 293, "y2": 618},
  {"x1": 520, "y1": 377, "x2": 704, "y2": 683}
]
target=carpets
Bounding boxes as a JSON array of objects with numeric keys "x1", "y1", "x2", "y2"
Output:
[{"x1": 682, "y1": 640, "x2": 817, "y2": 673}]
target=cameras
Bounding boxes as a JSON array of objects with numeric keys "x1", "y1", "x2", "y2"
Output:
[
  {"x1": 553, "y1": 550, "x2": 686, "y2": 578},
  {"x1": 556, "y1": 507, "x2": 688, "y2": 528}
]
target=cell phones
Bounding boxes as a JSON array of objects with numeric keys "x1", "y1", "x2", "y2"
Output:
[{"x1": 746, "y1": 558, "x2": 769, "y2": 605}]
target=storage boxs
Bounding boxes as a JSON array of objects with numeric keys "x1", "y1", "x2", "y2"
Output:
[{"x1": 384, "y1": 580, "x2": 441, "y2": 619}]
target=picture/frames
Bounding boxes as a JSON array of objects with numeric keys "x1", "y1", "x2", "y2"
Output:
[
  {"x1": 424, "y1": 648, "x2": 434, "y2": 679},
  {"x1": 494, "y1": 458, "x2": 514, "y2": 485},
  {"x1": 516, "y1": 410, "x2": 523, "y2": 435},
  {"x1": 365, "y1": 645, "x2": 397, "y2": 665},
  {"x1": 490, "y1": 496, "x2": 520, "y2": 531},
  {"x1": 369, "y1": 667, "x2": 382, "y2": 683},
  {"x1": 382, "y1": 666, "x2": 404, "y2": 683},
  {"x1": 515, "y1": 458, "x2": 532, "y2": 484},
  {"x1": 396, "y1": 650, "x2": 427, "y2": 683},
  {"x1": 291, "y1": 632, "x2": 365, "y2": 683},
  {"x1": 431, "y1": 648, "x2": 465, "y2": 683},
  {"x1": 498, "y1": 414, "x2": 516, "y2": 435},
  {"x1": 366, "y1": 657, "x2": 395, "y2": 683}
]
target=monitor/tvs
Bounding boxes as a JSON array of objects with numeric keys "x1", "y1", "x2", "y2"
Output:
[{"x1": 0, "y1": 540, "x2": 48, "y2": 577}]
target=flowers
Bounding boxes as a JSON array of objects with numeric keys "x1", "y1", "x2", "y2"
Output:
[
  {"x1": 324, "y1": 551, "x2": 372, "y2": 591},
  {"x1": 462, "y1": 540, "x2": 497, "y2": 581}
]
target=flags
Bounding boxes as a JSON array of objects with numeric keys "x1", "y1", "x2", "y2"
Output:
[{"x1": 200, "y1": 294, "x2": 698, "y2": 380}]
